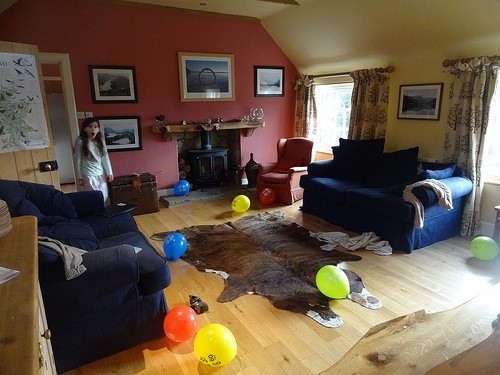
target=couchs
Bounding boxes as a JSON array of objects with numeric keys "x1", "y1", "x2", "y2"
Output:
[
  {"x1": 256, "y1": 136, "x2": 315, "y2": 204},
  {"x1": 0, "y1": 174, "x2": 172, "y2": 375},
  {"x1": 300, "y1": 146, "x2": 472, "y2": 254}
]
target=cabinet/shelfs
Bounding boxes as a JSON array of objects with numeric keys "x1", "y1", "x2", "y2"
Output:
[{"x1": 0, "y1": 213, "x2": 58, "y2": 375}]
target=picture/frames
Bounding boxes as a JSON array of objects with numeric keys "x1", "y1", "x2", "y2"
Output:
[
  {"x1": 177, "y1": 51, "x2": 237, "y2": 102},
  {"x1": 88, "y1": 65, "x2": 139, "y2": 104},
  {"x1": 253, "y1": 65, "x2": 286, "y2": 97},
  {"x1": 397, "y1": 82, "x2": 445, "y2": 120},
  {"x1": 95, "y1": 115, "x2": 143, "y2": 153}
]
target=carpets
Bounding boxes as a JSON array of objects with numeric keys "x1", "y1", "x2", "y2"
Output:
[{"x1": 145, "y1": 210, "x2": 384, "y2": 329}]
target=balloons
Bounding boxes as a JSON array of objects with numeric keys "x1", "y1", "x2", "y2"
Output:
[
  {"x1": 163, "y1": 306, "x2": 199, "y2": 342},
  {"x1": 163, "y1": 232, "x2": 187, "y2": 261},
  {"x1": 230, "y1": 194, "x2": 251, "y2": 212},
  {"x1": 316, "y1": 265, "x2": 350, "y2": 298},
  {"x1": 193, "y1": 323, "x2": 237, "y2": 367},
  {"x1": 470, "y1": 235, "x2": 500, "y2": 261},
  {"x1": 174, "y1": 180, "x2": 191, "y2": 197},
  {"x1": 260, "y1": 187, "x2": 276, "y2": 206}
]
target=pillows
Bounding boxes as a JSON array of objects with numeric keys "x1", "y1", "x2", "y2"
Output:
[
  {"x1": 376, "y1": 146, "x2": 422, "y2": 183},
  {"x1": 330, "y1": 145, "x2": 381, "y2": 180},
  {"x1": 392, "y1": 162, "x2": 458, "y2": 194},
  {"x1": 340, "y1": 136, "x2": 385, "y2": 154}
]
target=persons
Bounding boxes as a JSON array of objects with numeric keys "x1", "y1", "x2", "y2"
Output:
[{"x1": 74, "y1": 117, "x2": 114, "y2": 202}]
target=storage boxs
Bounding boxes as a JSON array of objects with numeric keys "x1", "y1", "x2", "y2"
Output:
[{"x1": 108, "y1": 172, "x2": 160, "y2": 216}]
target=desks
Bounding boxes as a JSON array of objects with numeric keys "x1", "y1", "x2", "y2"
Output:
[{"x1": 151, "y1": 118, "x2": 263, "y2": 136}]
